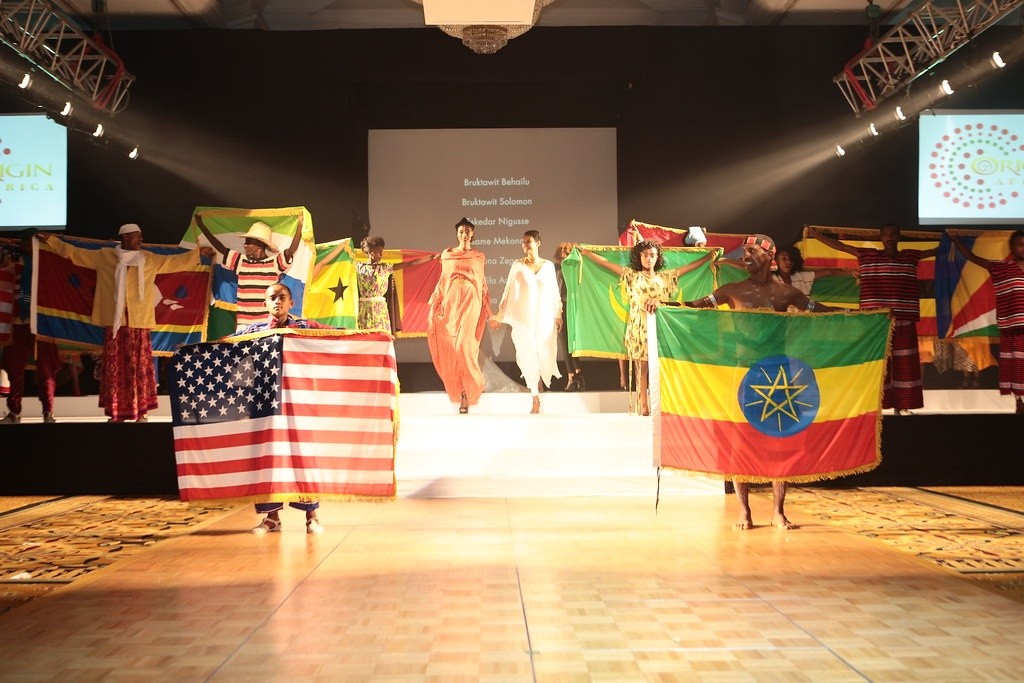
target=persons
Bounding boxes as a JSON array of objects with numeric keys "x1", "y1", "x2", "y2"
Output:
[
  {"x1": 427, "y1": 216, "x2": 495, "y2": 414},
  {"x1": 557, "y1": 226, "x2": 1024, "y2": 529},
  {"x1": 497, "y1": 230, "x2": 563, "y2": 414},
  {"x1": 0, "y1": 213, "x2": 440, "y2": 427},
  {"x1": 176, "y1": 285, "x2": 396, "y2": 535}
]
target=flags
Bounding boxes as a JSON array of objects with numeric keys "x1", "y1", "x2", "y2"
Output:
[
  {"x1": 302, "y1": 239, "x2": 358, "y2": 327},
  {"x1": 170, "y1": 333, "x2": 400, "y2": 502},
  {"x1": 179, "y1": 207, "x2": 317, "y2": 342},
  {"x1": 654, "y1": 307, "x2": 896, "y2": 484},
  {"x1": 0, "y1": 239, "x2": 85, "y2": 370},
  {"x1": 562, "y1": 243, "x2": 725, "y2": 357},
  {"x1": 30, "y1": 232, "x2": 218, "y2": 357},
  {"x1": 791, "y1": 224, "x2": 999, "y2": 370},
  {"x1": 354, "y1": 248, "x2": 444, "y2": 337},
  {"x1": 619, "y1": 220, "x2": 757, "y2": 289},
  {"x1": 936, "y1": 229, "x2": 1017, "y2": 342}
]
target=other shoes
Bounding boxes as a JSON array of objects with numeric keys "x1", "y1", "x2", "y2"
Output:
[
  {"x1": 136, "y1": 415, "x2": 147, "y2": 423},
  {"x1": 894, "y1": 409, "x2": 913, "y2": 415},
  {"x1": 107, "y1": 417, "x2": 124, "y2": 423}
]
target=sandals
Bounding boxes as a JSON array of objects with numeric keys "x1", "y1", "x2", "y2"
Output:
[
  {"x1": 306, "y1": 517, "x2": 322, "y2": 533},
  {"x1": 43, "y1": 411, "x2": 55, "y2": 423},
  {"x1": 250, "y1": 518, "x2": 282, "y2": 534},
  {"x1": 0, "y1": 413, "x2": 21, "y2": 424}
]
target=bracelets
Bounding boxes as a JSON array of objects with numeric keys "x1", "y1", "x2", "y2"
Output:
[{"x1": 678, "y1": 301, "x2": 685, "y2": 306}]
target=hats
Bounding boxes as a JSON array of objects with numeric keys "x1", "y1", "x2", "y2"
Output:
[
  {"x1": 119, "y1": 223, "x2": 142, "y2": 235},
  {"x1": 238, "y1": 222, "x2": 280, "y2": 253}
]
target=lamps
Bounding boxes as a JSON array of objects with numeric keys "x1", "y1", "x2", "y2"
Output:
[{"x1": 412, "y1": 0, "x2": 554, "y2": 54}]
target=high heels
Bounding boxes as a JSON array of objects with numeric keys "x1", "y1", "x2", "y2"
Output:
[
  {"x1": 620, "y1": 377, "x2": 636, "y2": 391},
  {"x1": 530, "y1": 400, "x2": 540, "y2": 414},
  {"x1": 460, "y1": 395, "x2": 467, "y2": 413},
  {"x1": 642, "y1": 406, "x2": 649, "y2": 416},
  {"x1": 565, "y1": 372, "x2": 585, "y2": 392}
]
